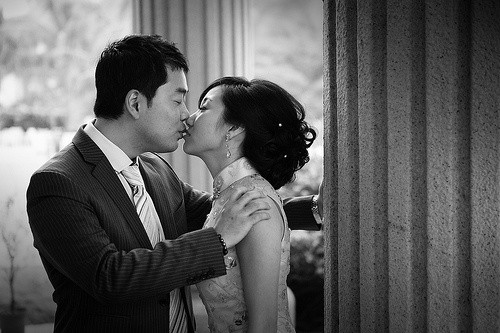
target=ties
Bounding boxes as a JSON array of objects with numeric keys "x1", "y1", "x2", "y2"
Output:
[{"x1": 122, "y1": 166, "x2": 189, "y2": 333}]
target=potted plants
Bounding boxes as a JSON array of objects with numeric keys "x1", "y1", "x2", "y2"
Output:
[{"x1": 0, "y1": 197, "x2": 30, "y2": 333}]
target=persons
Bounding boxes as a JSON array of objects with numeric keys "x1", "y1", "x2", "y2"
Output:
[
  {"x1": 25, "y1": 34, "x2": 331, "y2": 333},
  {"x1": 183, "y1": 75, "x2": 317, "y2": 333}
]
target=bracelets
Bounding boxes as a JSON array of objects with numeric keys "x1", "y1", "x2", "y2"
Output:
[{"x1": 312, "y1": 195, "x2": 323, "y2": 225}]
